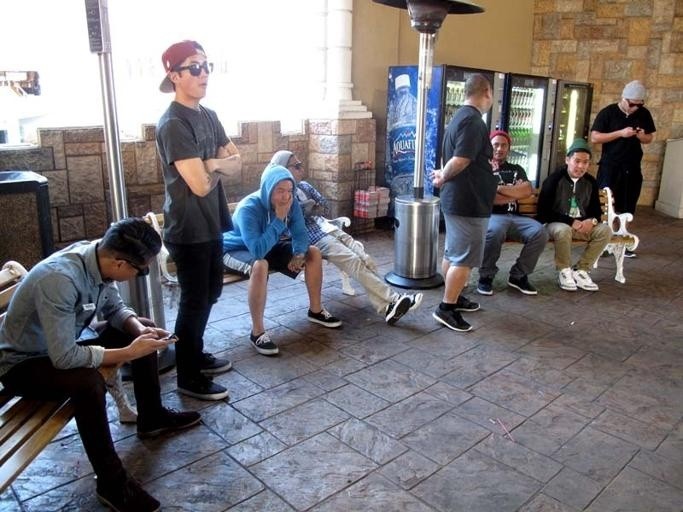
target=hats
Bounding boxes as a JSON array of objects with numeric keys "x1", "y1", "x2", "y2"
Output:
[
  {"x1": 566, "y1": 137, "x2": 592, "y2": 159},
  {"x1": 159, "y1": 40, "x2": 207, "y2": 93},
  {"x1": 489, "y1": 130, "x2": 511, "y2": 143},
  {"x1": 621, "y1": 80, "x2": 646, "y2": 100}
]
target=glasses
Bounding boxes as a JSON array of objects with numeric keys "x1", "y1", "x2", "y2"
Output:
[
  {"x1": 270, "y1": 150, "x2": 294, "y2": 168},
  {"x1": 115, "y1": 258, "x2": 150, "y2": 276},
  {"x1": 627, "y1": 100, "x2": 645, "y2": 108},
  {"x1": 178, "y1": 61, "x2": 214, "y2": 76},
  {"x1": 286, "y1": 161, "x2": 303, "y2": 169}
]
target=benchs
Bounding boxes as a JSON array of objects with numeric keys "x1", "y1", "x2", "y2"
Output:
[
  {"x1": 0, "y1": 260, "x2": 128, "y2": 512},
  {"x1": 507, "y1": 184, "x2": 640, "y2": 284},
  {"x1": 141, "y1": 203, "x2": 356, "y2": 311}
]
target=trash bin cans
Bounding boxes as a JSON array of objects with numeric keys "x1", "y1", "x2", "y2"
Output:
[{"x1": 0, "y1": 171, "x2": 53, "y2": 314}]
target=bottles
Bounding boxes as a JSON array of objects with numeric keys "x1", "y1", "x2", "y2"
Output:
[
  {"x1": 444, "y1": 86, "x2": 468, "y2": 129},
  {"x1": 389, "y1": 73, "x2": 417, "y2": 198},
  {"x1": 505, "y1": 86, "x2": 534, "y2": 177}
]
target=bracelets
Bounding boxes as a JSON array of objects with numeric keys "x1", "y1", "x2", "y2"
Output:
[{"x1": 592, "y1": 217, "x2": 599, "y2": 226}]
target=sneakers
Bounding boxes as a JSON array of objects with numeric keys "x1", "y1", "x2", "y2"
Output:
[
  {"x1": 555, "y1": 266, "x2": 579, "y2": 291},
  {"x1": 135, "y1": 407, "x2": 202, "y2": 440},
  {"x1": 176, "y1": 372, "x2": 229, "y2": 400},
  {"x1": 249, "y1": 330, "x2": 279, "y2": 355},
  {"x1": 94, "y1": 457, "x2": 161, "y2": 512},
  {"x1": 307, "y1": 307, "x2": 342, "y2": 328},
  {"x1": 382, "y1": 293, "x2": 480, "y2": 332},
  {"x1": 201, "y1": 353, "x2": 232, "y2": 374},
  {"x1": 507, "y1": 273, "x2": 538, "y2": 296},
  {"x1": 476, "y1": 277, "x2": 494, "y2": 296},
  {"x1": 571, "y1": 265, "x2": 600, "y2": 292},
  {"x1": 624, "y1": 249, "x2": 637, "y2": 258}
]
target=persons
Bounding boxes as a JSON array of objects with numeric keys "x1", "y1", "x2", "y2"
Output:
[
  {"x1": 156, "y1": 41, "x2": 242, "y2": 401},
  {"x1": 271, "y1": 149, "x2": 423, "y2": 326},
  {"x1": 475, "y1": 130, "x2": 548, "y2": 295},
  {"x1": 536, "y1": 138, "x2": 613, "y2": 292},
  {"x1": 0, "y1": 219, "x2": 202, "y2": 511},
  {"x1": 590, "y1": 80, "x2": 656, "y2": 258},
  {"x1": 427, "y1": 73, "x2": 495, "y2": 332},
  {"x1": 222, "y1": 163, "x2": 342, "y2": 355}
]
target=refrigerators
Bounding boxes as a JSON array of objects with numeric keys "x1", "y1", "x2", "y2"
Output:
[
  {"x1": 386, "y1": 63, "x2": 505, "y2": 218},
  {"x1": 500, "y1": 73, "x2": 556, "y2": 194}
]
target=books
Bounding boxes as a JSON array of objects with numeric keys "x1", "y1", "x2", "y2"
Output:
[{"x1": 353, "y1": 187, "x2": 390, "y2": 219}]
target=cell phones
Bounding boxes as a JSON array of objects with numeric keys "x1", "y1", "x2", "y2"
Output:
[
  {"x1": 154, "y1": 332, "x2": 175, "y2": 340},
  {"x1": 633, "y1": 130, "x2": 641, "y2": 133}
]
